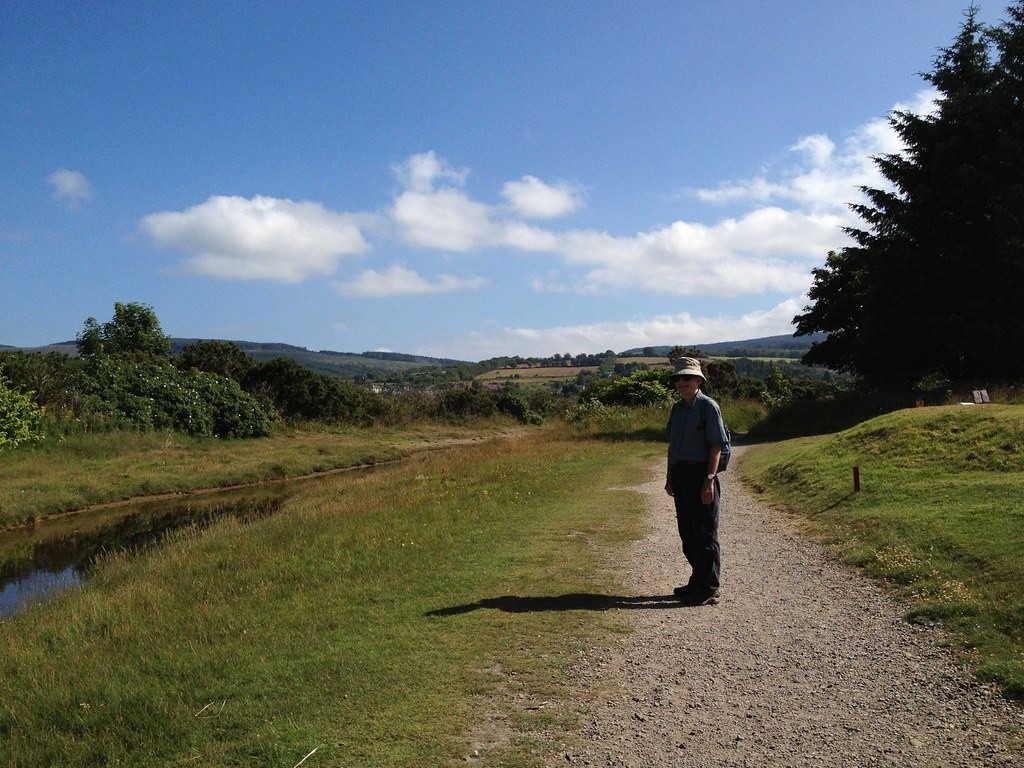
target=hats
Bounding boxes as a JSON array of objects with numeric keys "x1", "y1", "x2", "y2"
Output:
[{"x1": 669, "y1": 356, "x2": 709, "y2": 382}]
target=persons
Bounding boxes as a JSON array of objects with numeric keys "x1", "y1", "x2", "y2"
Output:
[{"x1": 664, "y1": 356, "x2": 728, "y2": 604}]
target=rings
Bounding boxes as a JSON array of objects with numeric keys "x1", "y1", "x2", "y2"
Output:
[{"x1": 707, "y1": 496, "x2": 710, "y2": 499}]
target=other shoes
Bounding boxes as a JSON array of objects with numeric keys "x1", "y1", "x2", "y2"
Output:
[{"x1": 673, "y1": 585, "x2": 721, "y2": 606}]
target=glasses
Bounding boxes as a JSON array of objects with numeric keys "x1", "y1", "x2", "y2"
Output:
[{"x1": 670, "y1": 374, "x2": 693, "y2": 383}]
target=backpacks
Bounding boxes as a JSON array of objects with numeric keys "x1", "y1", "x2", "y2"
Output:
[{"x1": 672, "y1": 397, "x2": 731, "y2": 473}]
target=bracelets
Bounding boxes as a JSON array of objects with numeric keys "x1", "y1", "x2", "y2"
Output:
[{"x1": 666, "y1": 476, "x2": 671, "y2": 479}]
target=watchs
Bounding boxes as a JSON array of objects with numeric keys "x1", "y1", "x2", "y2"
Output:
[{"x1": 704, "y1": 473, "x2": 716, "y2": 480}]
